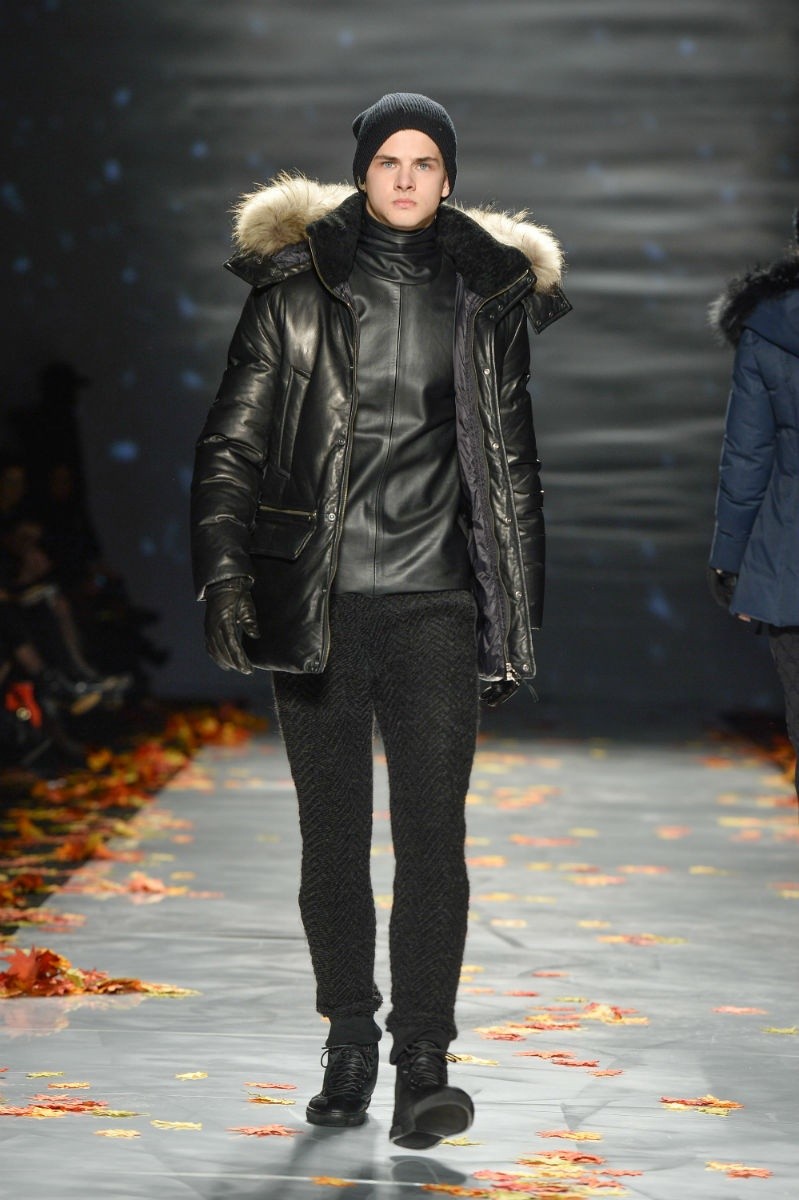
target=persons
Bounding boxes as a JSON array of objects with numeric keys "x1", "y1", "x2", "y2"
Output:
[
  {"x1": 0, "y1": 366, "x2": 178, "y2": 772},
  {"x1": 186, "y1": 92, "x2": 574, "y2": 1150},
  {"x1": 712, "y1": 258, "x2": 799, "y2": 803}
]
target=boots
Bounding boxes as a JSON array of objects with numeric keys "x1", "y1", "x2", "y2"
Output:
[
  {"x1": 389, "y1": 1040, "x2": 475, "y2": 1151},
  {"x1": 306, "y1": 1040, "x2": 379, "y2": 1126}
]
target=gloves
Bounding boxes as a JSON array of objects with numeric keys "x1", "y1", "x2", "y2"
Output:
[
  {"x1": 481, "y1": 681, "x2": 521, "y2": 707},
  {"x1": 707, "y1": 566, "x2": 738, "y2": 609},
  {"x1": 204, "y1": 578, "x2": 260, "y2": 676}
]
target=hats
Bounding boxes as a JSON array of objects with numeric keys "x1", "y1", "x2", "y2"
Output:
[{"x1": 351, "y1": 92, "x2": 457, "y2": 205}]
